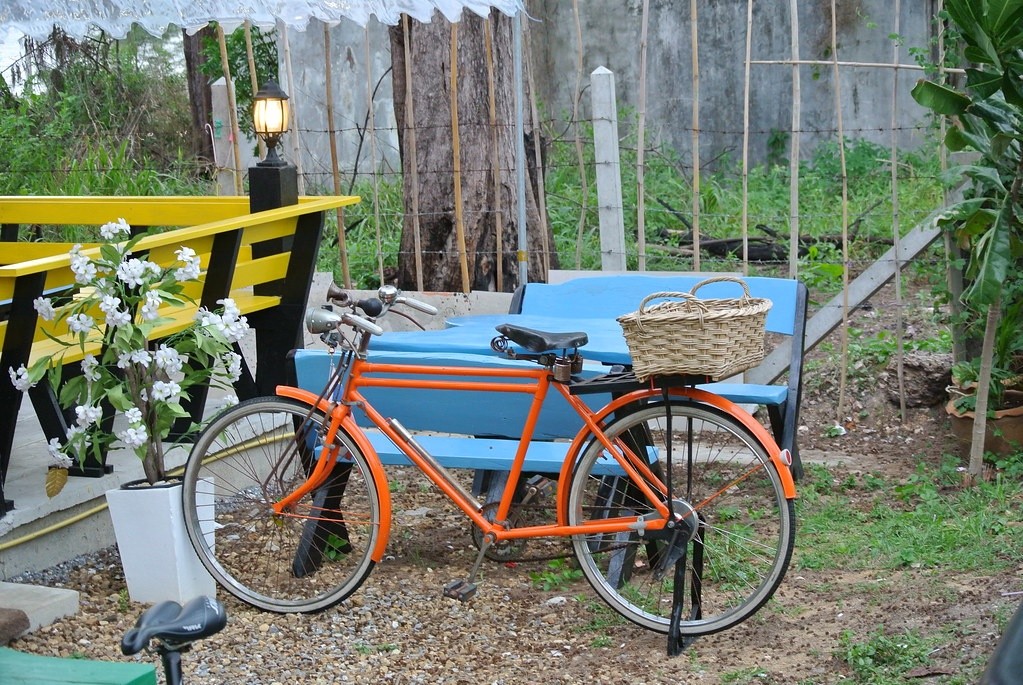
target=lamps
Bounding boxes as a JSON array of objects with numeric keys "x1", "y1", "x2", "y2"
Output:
[{"x1": 251, "y1": 75, "x2": 296, "y2": 169}]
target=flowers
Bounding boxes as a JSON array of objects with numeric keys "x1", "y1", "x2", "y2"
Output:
[{"x1": 4, "y1": 217, "x2": 256, "y2": 485}]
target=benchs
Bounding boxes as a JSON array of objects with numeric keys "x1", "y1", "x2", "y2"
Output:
[
  {"x1": 285, "y1": 348, "x2": 675, "y2": 594},
  {"x1": 0, "y1": 193, "x2": 366, "y2": 523},
  {"x1": 471, "y1": 274, "x2": 813, "y2": 482}
]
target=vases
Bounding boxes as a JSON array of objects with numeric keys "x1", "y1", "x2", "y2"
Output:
[{"x1": 100, "y1": 475, "x2": 219, "y2": 609}]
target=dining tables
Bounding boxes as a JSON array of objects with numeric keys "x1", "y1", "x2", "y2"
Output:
[{"x1": 361, "y1": 314, "x2": 665, "y2": 520}]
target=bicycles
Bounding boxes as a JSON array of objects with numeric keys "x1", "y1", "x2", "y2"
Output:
[{"x1": 176, "y1": 280, "x2": 801, "y2": 662}]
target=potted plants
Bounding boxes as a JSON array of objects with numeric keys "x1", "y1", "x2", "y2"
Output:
[
  {"x1": 945, "y1": 272, "x2": 1023, "y2": 394},
  {"x1": 941, "y1": 303, "x2": 1023, "y2": 471}
]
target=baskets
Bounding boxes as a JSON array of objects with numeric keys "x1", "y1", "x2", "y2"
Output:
[{"x1": 613, "y1": 270, "x2": 771, "y2": 387}]
